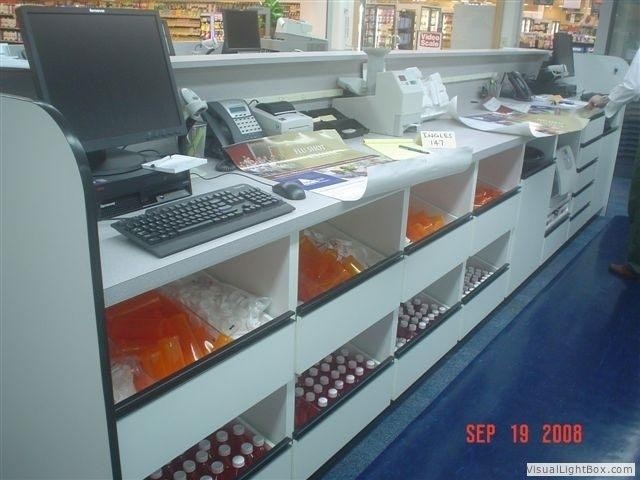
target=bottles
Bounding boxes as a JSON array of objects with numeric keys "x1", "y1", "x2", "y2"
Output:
[
  {"x1": 151, "y1": 425, "x2": 267, "y2": 480},
  {"x1": 397, "y1": 298, "x2": 446, "y2": 350},
  {"x1": 461, "y1": 266, "x2": 494, "y2": 297},
  {"x1": 294, "y1": 345, "x2": 378, "y2": 427}
]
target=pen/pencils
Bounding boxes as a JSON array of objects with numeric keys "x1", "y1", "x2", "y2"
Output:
[
  {"x1": 151, "y1": 154, "x2": 171, "y2": 168},
  {"x1": 399, "y1": 145, "x2": 430, "y2": 154}
]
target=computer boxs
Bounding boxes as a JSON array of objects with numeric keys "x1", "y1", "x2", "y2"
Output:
[
  {"x1": 526, "y1": 80, "x2": 576, "y2": 98},
  {"x1": 92, "y1": 153, "x2": 192, "y2": 222}
]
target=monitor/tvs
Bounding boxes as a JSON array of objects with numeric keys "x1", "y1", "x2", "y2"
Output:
[
  {"x1": 221, "y1": 9, "x2": 262, "y2": 54},
  {"x1": 15, "y1": 5, "x2": 189, "y2": 177},
  {"x1": 544, "y1": 33, "x2": 575, "y2": 81}
]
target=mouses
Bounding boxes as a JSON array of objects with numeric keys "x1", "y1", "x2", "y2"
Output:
[{"x1": 273, "y1": 181, "x2": 306, "y2": 200}]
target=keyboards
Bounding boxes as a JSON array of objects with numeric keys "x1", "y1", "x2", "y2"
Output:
[{"x1": 111, "y1": 184, "x2": 296, "y2": 259}]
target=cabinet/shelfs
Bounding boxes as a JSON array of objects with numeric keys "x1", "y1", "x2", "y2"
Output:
[
  {"x1": 1, "y1": 12, "x2": 22, "y2": 44},
  {"x1": 570, "y1": 112, "x2": 619, "y2": 239},
  {"x1": 366, "y1": 3, "x2": 453, "y2": 51},
  {"x1": 509, "y1": 163, "x2": 556, "y2": 296},
  {"x1": 155, "y1": 0, "x2": 302, "y2": 40},
  {"x1": 101, "y1": 184, "x2": 519, "y2": 479}
]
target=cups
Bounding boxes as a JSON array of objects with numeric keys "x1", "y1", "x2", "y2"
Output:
[
  {"x1": 300, "y1": 230, "x2": 364, "y2": 302},
  {"x1": 102, "y1": 291, "x2": 230, "y2": 394},
  {"x1": 407, "y1": 207, "x2": 445, "y2": 242}
]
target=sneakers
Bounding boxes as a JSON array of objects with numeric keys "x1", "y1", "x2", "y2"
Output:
[{"x1": 608, "y1": 262, "x2": 640, "y2": 280}]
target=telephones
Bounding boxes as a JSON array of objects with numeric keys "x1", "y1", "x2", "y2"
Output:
[
  {"x1": 507, "y1": 70, "x2": 534, "y2": 101},
  {"x1": 202, "y1": 98, "x2": 266, "y2": 146}
]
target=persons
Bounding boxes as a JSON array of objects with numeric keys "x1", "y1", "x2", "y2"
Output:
[{"x1": 588, "y1": 47, "x2": 640, "y2": 281}]
